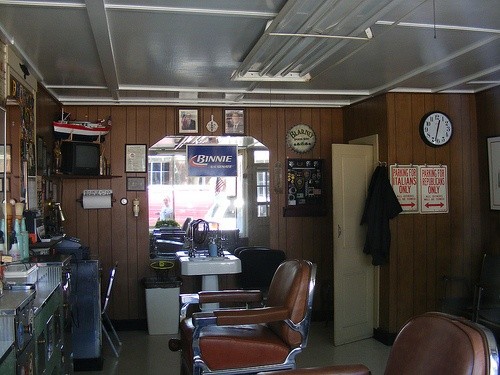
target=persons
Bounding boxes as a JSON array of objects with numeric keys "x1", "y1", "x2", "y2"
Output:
[
  {"x1": 182, "y1": 114, "x2": 195, "y2": 130},
  {"x1": 226, "y1": 112, "x2": 243, "y2": 133}
]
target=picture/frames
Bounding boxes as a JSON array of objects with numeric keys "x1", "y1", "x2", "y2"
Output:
[
  {"x1": 222, "y1": 107, "x2": 247, "y2": 136},
  {"x1": 175, "y1": 106, "x2": 200, "y2": 136}
]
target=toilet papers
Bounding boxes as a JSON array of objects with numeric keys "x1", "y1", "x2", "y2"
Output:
[{"x1": 82, "y1": 194, "x2": 111, "y2": 209}]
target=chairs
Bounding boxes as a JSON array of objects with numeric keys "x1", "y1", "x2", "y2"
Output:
[
  {"x1": 180, "y1": 256, "x2": 318, "y2": 375},
  {"x1": 260, "y1": 312, "x2": 499, "y2": 375}
]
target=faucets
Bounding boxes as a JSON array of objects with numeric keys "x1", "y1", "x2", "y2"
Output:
[{"x1": 187, "y1": 218, "x2": 210, "y2": 258}]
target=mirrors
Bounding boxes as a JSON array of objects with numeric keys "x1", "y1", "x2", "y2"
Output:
[{"x1": 0, "y1": 65, "x2": 38, "y2": 255}]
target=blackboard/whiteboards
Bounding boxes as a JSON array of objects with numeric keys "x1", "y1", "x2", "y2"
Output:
[{"x1": 286, "y1": 158, "x2": 324, "y2": 209}]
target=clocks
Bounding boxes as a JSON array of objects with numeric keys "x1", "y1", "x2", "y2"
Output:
[{"x1": 419, "y1": 111, "x2": 453, "y2": 148}]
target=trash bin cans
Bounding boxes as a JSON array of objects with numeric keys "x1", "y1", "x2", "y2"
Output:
[{"x1": 143, "y1": 278, "x2": 181, "y2": 334}]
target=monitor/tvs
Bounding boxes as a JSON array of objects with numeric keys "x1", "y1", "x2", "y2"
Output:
[{"x1": 60, "y1": 141, "x2": 100, "y2": 175}]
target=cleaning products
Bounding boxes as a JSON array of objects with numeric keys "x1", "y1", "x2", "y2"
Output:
[{"x1": 209, "y1": 237, "x2": 217, "y2": 256}]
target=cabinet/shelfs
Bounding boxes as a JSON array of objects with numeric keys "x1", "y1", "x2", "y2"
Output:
[{"x1": 0, "y1": 277, "x2": 75, "y2": 375}]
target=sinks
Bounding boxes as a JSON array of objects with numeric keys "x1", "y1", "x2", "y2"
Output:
[{"x1": 178, "y1": 255, "x2": 242, "y2": 275}]
